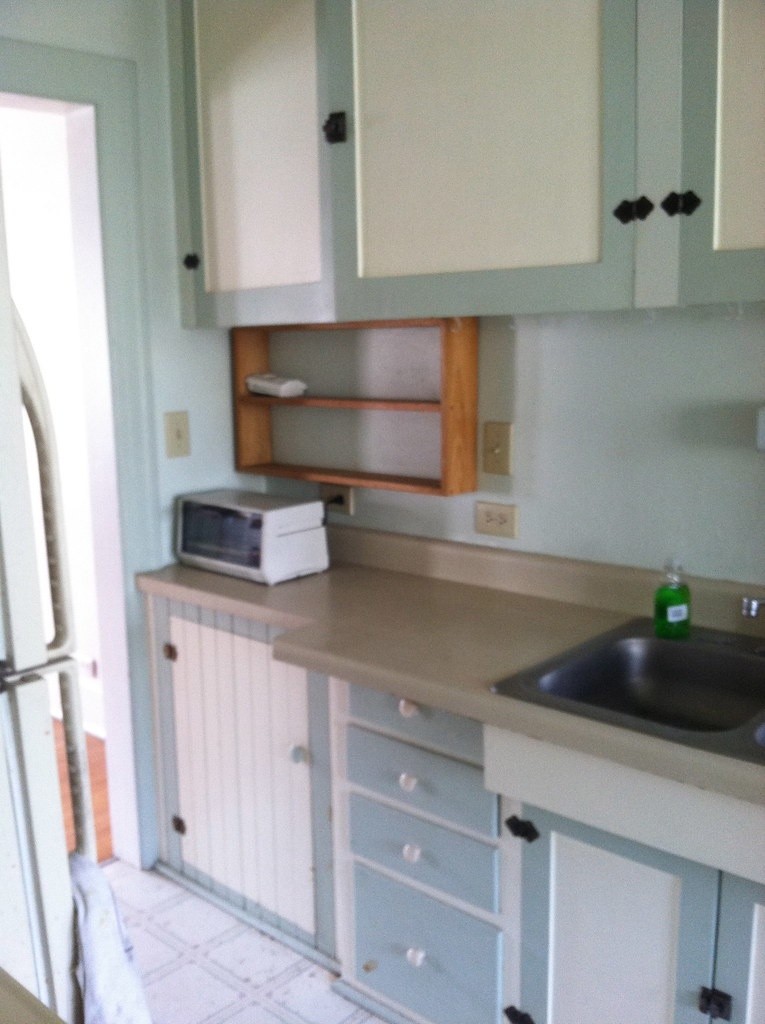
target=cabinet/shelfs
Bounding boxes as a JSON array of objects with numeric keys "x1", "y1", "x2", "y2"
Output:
[
  {"x1": 183, "y1": 0, "x2": 338, "y2": 327},
  {"x1": 498, "y1": 796, "x2": 764, "y2": 1024},
  {"x1": 339, "y1": 0, "x2": 765, "y2": 321},
  {"x1": 231, "y1": 318, "x2": 477, "y2": 495},
  {"x1": 144, "y1": 593, "x2": 332, "y2": 967},
  {"x1": 330, "y1": 673, "x2": 499, "y2": 1024}
]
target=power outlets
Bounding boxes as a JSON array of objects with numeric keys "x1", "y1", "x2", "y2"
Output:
[
  {"x1": 320, "y1": 485, "x2": 352, "y2": 516},
  {"x1": 475, "y1": 502, "x2": 518, "y2": 540}
]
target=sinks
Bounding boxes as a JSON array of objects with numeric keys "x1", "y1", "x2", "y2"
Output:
[
  {"x1": 488, "y1": 611, "x2": 765, "y2": 760},
  {"x1": 745, "y1": 722, "x2": 765, "y2": 766}
]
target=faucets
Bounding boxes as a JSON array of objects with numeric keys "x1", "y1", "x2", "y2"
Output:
[{"x1": 741, "y1": 597, "x2": 765, "y2": 619}]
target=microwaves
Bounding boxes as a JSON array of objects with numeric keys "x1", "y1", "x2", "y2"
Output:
[{"x1": 176, "y1": 489, "x2": 329, "y2": 587}]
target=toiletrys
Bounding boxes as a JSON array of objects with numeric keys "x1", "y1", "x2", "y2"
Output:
[{"x1": 653, "y1": 559, "x2": 691, "y2": 639}]
